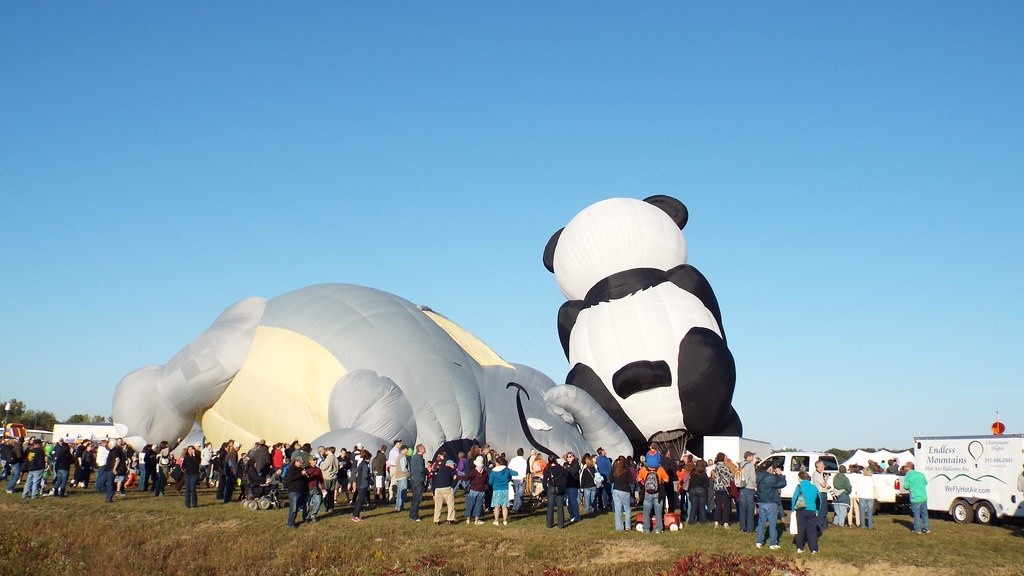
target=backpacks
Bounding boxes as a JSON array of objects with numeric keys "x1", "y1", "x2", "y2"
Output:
[
  {"x1": 733, "y1": 462, "x2": 751, "y2": 488},
  {"x1": 643, "y1": 466, "x2": 659, "y2": 494},
  {"x1": 6, "y1": 450, "x2": 17, "y2": 464}
]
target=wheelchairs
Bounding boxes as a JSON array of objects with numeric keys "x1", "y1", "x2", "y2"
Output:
[{"x1": 239, "y1": 484, "x2": 280, "y2": 510}]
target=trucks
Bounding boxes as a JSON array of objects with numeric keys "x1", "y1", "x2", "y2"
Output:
[{"x1": 914, "y1": 434, "x2": 1024, "y2": 525}]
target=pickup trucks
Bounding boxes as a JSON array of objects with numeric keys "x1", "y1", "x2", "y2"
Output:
[{"x1": 755, "y1": 451, "x2": 910, "y2": 516}]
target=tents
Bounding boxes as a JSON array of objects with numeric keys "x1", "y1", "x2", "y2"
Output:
[{"x1": 840, "y1": 450, "x2": 915, "y2": 470}]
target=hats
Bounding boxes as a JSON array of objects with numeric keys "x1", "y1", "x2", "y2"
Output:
[
  {"x1": 294, "y1": 456, "x2": 305, "y2": 463},
  {"x1": 309, "y1": 455, "x2": 319, "y2": 460},
  {"x1": 473, "y1": 455, "x2": 485, "y2": 467},
  {"x1": 19, "y1": 437, "x2": 25, "y2": 442},
  {"x1": 393, "y1": 439, "x2": 402, "y2": 445},
  {"x1": 743, "y1": 451, "x2": 756, "y2": 459}
]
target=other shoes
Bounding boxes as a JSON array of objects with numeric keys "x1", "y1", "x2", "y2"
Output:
[
  {"x1": 312, "y1": 516, "x2": 317, "y2": 522},
  {"x1": 922, "y1": 528, "x2": 931, "y2": 533},
  {"x1": 769, "y1": 544, "x2": 781, "y2": 550},
  {"x1": 756, "y1": 542, "x2": 765, "y2": 548},
  {"x1": 810, "y1": 549, "x2": 821, "y2": 555},
  {"x1": 466, "y1": 520, "x2": 484, "y2": 525},
  {"x1": 493, "y1": 520, "x2": 507, "y2": 526},
  {"x1": 911, "y1": 530, "x2": 921, "y2": 535},
  {"x1": 796, "y1": 548, "x2": 806, "y2": 554},
  {"x1": 116, "y1": 491, "x2": 127, "y2": 497},
  {"x1": 352, "y1": 516, "x2": 363, "y2": 522},
  {"x1": 6, "y1": 489, "x2": 16, "y2": 494}
]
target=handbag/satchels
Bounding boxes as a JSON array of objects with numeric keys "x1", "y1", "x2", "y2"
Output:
[
  {"x1": 794, "y1": 493, "x2": 807, "y2": 510},
  {"x1": 464, "y1": 484, "x2": 472, "y2": 493},
  {"x1": 375, "y1": 475, "x2": 385, "y2": 489},
  {"x1": 507, "y1": 481, "x2": 515, "y2": 501},
  {"x1": 789, "y1": 510, "x2": 799, "y2": 534},
  {"x1": 170, "y1": 467, "x2": 183, "y2": 482},
  {"x1": 554, "y1": 486, "x2": 567, "y2": 496}
]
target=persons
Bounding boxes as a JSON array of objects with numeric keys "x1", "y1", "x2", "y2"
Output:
[
  {"x1": 756, "y1": 461, "x2": 787, "y2": 549},
  {"x1": 812, "y1": 461, "x2": 879, "y2": 530},
  {"x1": 1017, "y1": 463, "x2": 1024, "y2": 497},
  {"x1": 0, "y1": 435, "x2": 387, "y2": 508},
  {"x1": 385, "y1": 439, "x2": 785, "y2": 534},
  {"x1": 286, "y1": 456, "x2": 326, "y2": 527},
  {"x1": 351, "y1": 453, "x2": 374, "y2": 522},
  {"x1": 868, "y1": 457, "x2": 908, "y2": 476},
  {"x1": 903, "y1": 462, "x2": 930, "y2": 534},
  {"x1": 801, "y1": 461, "x2": 807, "y2": 471},
  {"x1": 791, "y1": 471, "x2": 822, "y2": 554}
]
